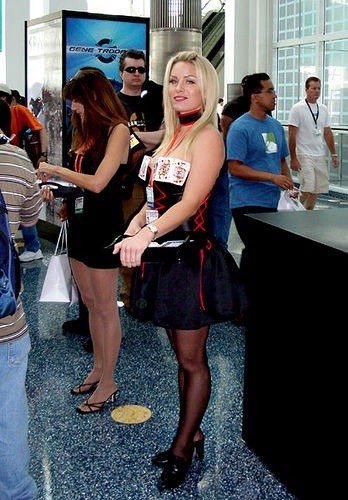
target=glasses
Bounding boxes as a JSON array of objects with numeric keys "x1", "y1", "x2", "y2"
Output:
[
  {"x1": 262, "y1": 90, "x2": 275, "y2": 95},
  {"x1": 123, "y1": 66, "x2": 146, "y2": 74}
]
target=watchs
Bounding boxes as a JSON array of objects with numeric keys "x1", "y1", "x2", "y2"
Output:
[
  {"x1": 41, "y1": 152, "x2": 47, "y2": 157},
  {"x1": 147, "y1": 224, "x2": 158, "y2": 240}
]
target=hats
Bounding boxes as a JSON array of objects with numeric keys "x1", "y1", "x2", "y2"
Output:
[{"x1": 0, "y1": 83, "x2": 12, "y2": 95}]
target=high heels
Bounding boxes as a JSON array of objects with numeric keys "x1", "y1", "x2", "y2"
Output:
[
  {"x1": 151, "y1": 434, "x2": 204, "y2": 467},
  {"x1": 70, "y1": 378, "x2": 100, "y2": 396},
  {"x1": 157, "y1": 447, "x2": 195, "y2": 491},
  {"x1": 76, "y1": 390, "x2": 118, "y2": 414}
]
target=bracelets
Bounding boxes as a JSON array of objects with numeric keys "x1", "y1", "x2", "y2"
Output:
[{"x1": 331, "y1": 154, "x2": 337, "y2": 156}]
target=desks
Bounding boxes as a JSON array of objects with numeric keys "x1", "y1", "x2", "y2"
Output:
[{"x1": 235, "y1": 204, "x2": 348, "y2": 500}]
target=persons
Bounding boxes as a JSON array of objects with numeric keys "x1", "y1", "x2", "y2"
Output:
[
  {"x1": 38, "y1": 66, "x2": 132, "y2": 414},
  {"x1": 220, "y1": 76, "x2": 274, "y2": 149},
  {"x1": 288, "y1": 77, "x2": 339, "y2": 210},
  {"x1": 0, "y1": 192, "x2": 38, "y2": 500},
  {"x1": 115, "y1": 50, "x2": 166, "y2": 313},
  {"x1": 227, "y1": 73, "x2": 293, "y2": 268},
  {"x1": 217, "y1": 98, "x2": 224, "y2": 132},
  {"x1": 113, "y1": 51, "x2": 247, "y2": 491},
  {"x1": 0, "y1": 83, "x2": 47, "y2": 261}
]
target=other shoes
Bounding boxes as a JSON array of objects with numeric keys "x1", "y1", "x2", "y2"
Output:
[
  {"x1": 82, "y1": 337, "x2": 93, "y2": 353},
  {"x1": 61, "y1": 319, "x2": 89, "y2": 331}
]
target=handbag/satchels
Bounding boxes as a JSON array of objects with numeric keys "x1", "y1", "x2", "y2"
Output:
[
  {"x1": 38, "y1": 220, "x2": 78, "y2": 307},
  {"x1": 0, "y1": 268, "x2": 17, "y2": 319},
  {"x1": 277, "y1": 187, "x2": 307, "y2": 212}
]
